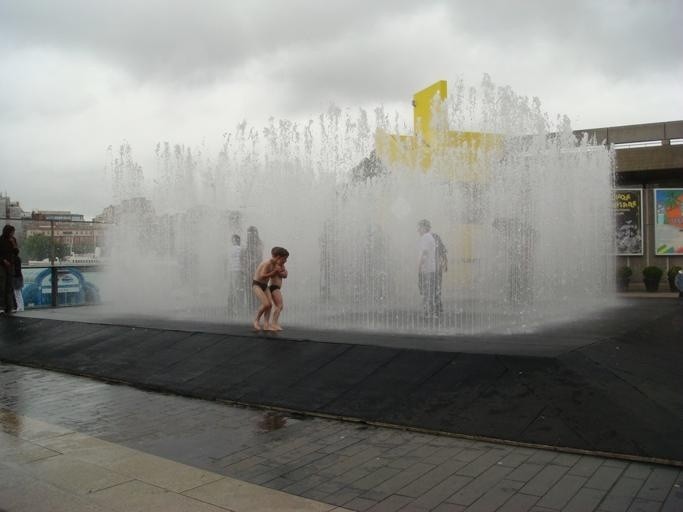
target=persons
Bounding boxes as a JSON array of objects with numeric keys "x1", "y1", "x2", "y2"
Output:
[
  {"x1": 223, "y1": 234, "x2": 243, "y2": 317},
  {"x1": 255, "y1": 411, "x2": 283, "y2": 434},
  {"x1": 0, "y1": 359, "x2": 21, "y2": 440},
  {"x1": 411, "y1": 221, "x2": 435, "y2": 319},
  {"x1": 239, "y1": 224, "x2": 265, "y2": 310},
  {"x1": 268, "y1": 248, "x2": 289, "y2": 330},
  {"x1": 0, "y1": 224, "x2": 17, "y2": 315},
  {"x1": 250, "y1": 246, "x2": 281, "y2": 332},
  {"x1": 3, "y1": 236, "x2": 24, "y2": 311},
  {"x1": 419, "y1": 219, "x2": 448, "y2": 316}
]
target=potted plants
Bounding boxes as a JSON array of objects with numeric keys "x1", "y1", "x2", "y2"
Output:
[
  {"x1": 617, "y1": 267, "x2": 632, "y2": 292},
  {"x1": 667, "y1": 266, "x2": 683, "y2": 293},
  {"x1": 643, "y1": 266, "x2": 662, "y2": 292}
]
